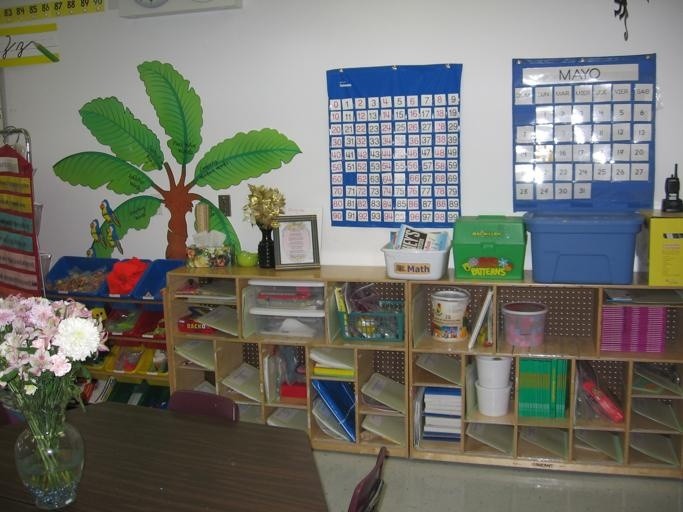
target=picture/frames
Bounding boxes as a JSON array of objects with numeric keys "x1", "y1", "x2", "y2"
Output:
[{"x1": 272, "y1": 214, "x2": 321, "y2": 271}]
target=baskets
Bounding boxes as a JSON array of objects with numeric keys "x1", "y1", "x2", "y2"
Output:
[{"x1": 336, "y1": 300, "x2": 403, "y2": 342}]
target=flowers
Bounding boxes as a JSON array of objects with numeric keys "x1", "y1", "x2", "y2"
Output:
[
  {"x1": 0, "y1": 292, "x2": 110, "y2": 488},
  {"x1": 242, "y1": 183, "x2": 288, "y2": 231}
]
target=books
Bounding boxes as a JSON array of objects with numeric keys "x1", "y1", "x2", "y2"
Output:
[
  {"x1": 171, "y1": 278, "x2": 239, "y2": 338},
  {"x1": 516, "y1": 288, "x2": 682, "y2": 434},
  {"x1": 628, "y1": 436, "x2": 681, "y2": 470},
  {"x1": 465, "y1": 424, "x2": 513, "y2": 456},
  {"x1": 174, "y1": 337, "x2": 461, "y2": 451},
  {"x1": 485, "y1": 295, "x2": 494, "y2": 348},
  {"x1": 384, "y1": 222, "x2": 453, "y2": 252},
  {"x1": 467, "y1": 285, "x2": 495, "y2": 351},
  {"x1": 520, "y1": 425, "x2": 568, "y2": 460},
  {"x1": 65, "y1": 372, "x2": 172, "y2": 412},
  {"x1": 572, "y1": 429, "x2": 624, "y2": 466}
]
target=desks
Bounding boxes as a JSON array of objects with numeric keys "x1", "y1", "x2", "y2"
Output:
[{"x1": 1, "y1": 399, "x2": 330, "y2": 511}]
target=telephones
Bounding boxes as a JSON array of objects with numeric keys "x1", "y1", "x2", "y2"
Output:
[{"x1": 661, "y1": 164, "x2": 683, "y2": 212}]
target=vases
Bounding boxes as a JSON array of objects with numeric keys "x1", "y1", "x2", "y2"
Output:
[{"x1": 257, "y1": 225, "x2": 276, "y2": 269}]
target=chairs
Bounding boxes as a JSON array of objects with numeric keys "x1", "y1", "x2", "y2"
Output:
[{"x1": 346, "y1": 446, "x2": 390, "y2": 511}]
